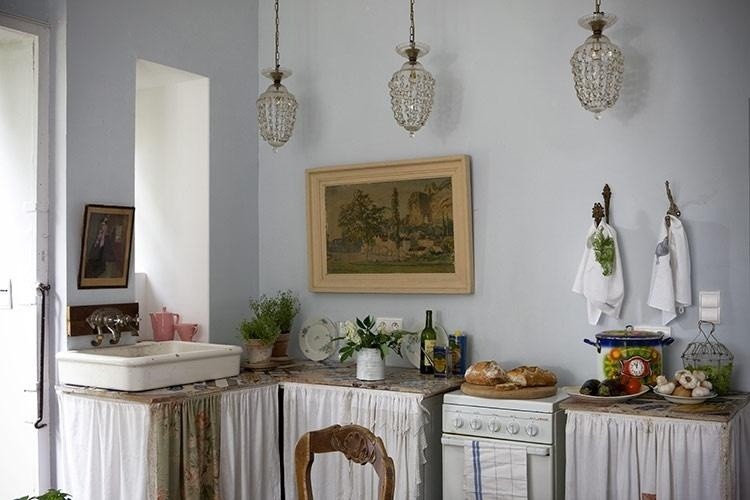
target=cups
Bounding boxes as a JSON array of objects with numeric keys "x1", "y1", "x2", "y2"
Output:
[
  {"x1": 431, "y1": 330, "x2": 466, "y2": 377},
  {"x1": 178, "y1": 323, "x2": 200, "y2": 341}
]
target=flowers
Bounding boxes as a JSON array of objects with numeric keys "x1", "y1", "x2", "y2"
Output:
[{"x1": 329, "y1": 315, "x2": 418, "y2": 360}]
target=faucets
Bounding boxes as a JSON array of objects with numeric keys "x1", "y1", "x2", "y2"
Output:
[{"x1": 91, "y1": 310, "x2": 143, "y2": 345}]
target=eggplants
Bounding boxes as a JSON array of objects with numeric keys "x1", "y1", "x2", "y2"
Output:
[{"x1": 579, "y1": 379, "x2": 621, "y2": 397}]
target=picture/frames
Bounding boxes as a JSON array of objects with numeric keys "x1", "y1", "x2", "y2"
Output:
[
  {"x1": 75, "y1": 202, "x2": 135, "y2": 290},
  {"x1": 304, "y1": 153, "x2": 475, "y2": 296}
]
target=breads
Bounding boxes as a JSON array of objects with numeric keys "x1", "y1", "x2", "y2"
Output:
[{"x1": 464, "y1": 360, "x2": 557, "y2": 391}]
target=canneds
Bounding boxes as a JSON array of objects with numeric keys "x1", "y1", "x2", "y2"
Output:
[{"x1": 448, "y1": 334, "x2": 467, "y2": 377}]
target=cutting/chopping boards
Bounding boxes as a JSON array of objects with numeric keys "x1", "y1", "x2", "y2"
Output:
[{"x1": 460, "y1": 381, "x2": 558, "y2": 400}]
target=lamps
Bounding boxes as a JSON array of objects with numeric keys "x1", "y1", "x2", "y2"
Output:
[
  {"x1": 253, "y1": 0, "x2": 297, "y2": 154},
  {"x1": 570, "y1": 1, "x2": 629, "y2": 120},
  {"x1": 386, "y1": 0, "x2": 439, "y2": 140}
]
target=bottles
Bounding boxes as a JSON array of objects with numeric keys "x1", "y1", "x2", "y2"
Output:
[{"x1": 417, "y1": 308, "x2": 435, "y2": 374}]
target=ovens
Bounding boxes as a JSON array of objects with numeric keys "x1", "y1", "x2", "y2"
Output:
[{"x1": 440, "y1": 404, "x2": 565, "y2": 499}]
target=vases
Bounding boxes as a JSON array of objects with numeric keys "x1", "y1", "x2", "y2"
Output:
[{"x1": 356, "y1": 344, "x2": 386, "y2": 381}]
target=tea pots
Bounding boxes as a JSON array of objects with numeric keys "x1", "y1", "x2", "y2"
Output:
[{"x1": 148, "y1": 305, "x2": 179, "y2": 342}]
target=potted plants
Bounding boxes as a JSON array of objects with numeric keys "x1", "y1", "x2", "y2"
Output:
[{"x1": 239, "y1": 285, "x2": 298, "y2": 372}]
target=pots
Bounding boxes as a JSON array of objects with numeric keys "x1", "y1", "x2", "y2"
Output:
[{"x1": 583, "y1": 325, "x2": 676, "y2": 386}]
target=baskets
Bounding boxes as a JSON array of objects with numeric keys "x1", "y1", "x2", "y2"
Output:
[{"x1": 682, "y1": 321, "x2": 734, "y2": 396}]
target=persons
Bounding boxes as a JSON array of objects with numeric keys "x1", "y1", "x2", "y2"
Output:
[{"x1": 84, "y1": 218, "x2": 118, "y2": 280}]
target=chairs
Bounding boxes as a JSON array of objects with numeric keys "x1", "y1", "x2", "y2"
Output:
[{"x1": 295, "y1": 424, "x2": 395, "y2": 500}]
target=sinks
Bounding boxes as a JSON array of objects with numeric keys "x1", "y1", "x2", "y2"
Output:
[{"x1": 55, "y1": 339, "x2": 242, "y2": 395}]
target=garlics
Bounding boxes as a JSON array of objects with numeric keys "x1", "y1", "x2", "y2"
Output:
[{"x1": 656, "y1": 369, "x2": 713, "y2": 398}]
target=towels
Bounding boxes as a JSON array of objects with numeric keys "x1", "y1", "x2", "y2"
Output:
[
  {"x1": 646, "y1": 214, "x2": 695, "y2": 325},
  {"x1": 573, "y1": 220, "x2": 625, "y2": 326},
  {"x1": 463, "y1": 439, "x2": 530, "y2": 500}
]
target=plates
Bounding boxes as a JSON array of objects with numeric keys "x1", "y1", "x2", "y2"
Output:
[
  {"x1": 565, "y1": 385, "x2": 650, "y2": 403},
  {"x1": 653, "y1": 386, "x2": 718, "y2": 405},
  {"x1": 298, "y1": 313, "x2": 338, "y2": 362},
  {"x1": 404, "y1": 320, "x2": 450, "y2": 369}
]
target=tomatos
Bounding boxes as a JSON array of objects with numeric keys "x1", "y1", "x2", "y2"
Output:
[{"x1": 625, "y1": 379, "x2": 641, "y2": 394}]
